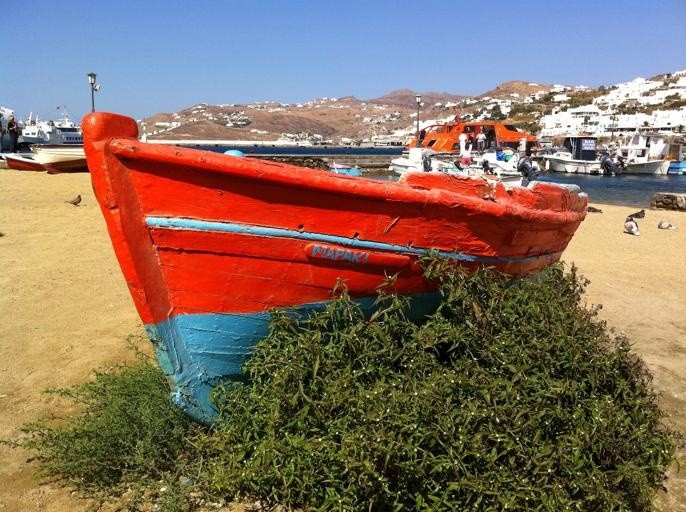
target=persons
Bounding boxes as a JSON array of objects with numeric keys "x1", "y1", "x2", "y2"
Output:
[
  {"x1": 483, "y1": 160, "x2": 497, "y2": 175},
  {"x1": 476, "y1": 130, "x2": 487, "y2": 153},
  {"x1": 467, "y1": 132, "x2": 474, "y2": 155},
  {"x1": 0, "y1": 114, "x2": 3, "y2": 153},
  {"x1": 423, "y1": 155, "x2": 433, "y2": 172},
  {"x1": 454, "y1": 158, "x2": 464, "y2": 171},
  {"x1": 8, "y1": 116, "x2": 18, "y2": 153},
  {"x1": 458, "y1": 131, "x2": 467, "y2": 156}
]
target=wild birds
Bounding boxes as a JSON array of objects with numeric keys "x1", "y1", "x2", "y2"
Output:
[
  {"x1": 66, "y1": 194, "x2": 82, "y2": 206},
  {"x1": 658, "y1": 220, "x2": 672, "y2": 230},
  {"x1": 624, "y1": 217, "x2": 641, "y2": 236},
  {"x1": 587, "y1": 206, "x2": 603, "y2": 214},
  {"x1": 628, "y1": 209, "x2": 645, "y2": 219}
]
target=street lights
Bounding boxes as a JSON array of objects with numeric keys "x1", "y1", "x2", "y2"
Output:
[
  {"x1": 86, "y1": 71, "x2": 98, "y2": 113},
  {"x1": 414, "y1": 92, "x2": 423, "y2": 133}
]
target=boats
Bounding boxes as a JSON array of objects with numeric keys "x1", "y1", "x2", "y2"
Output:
[
  {"x1": 388, "y1": 110, "x2": 686, "y2": 184},
  {"x1": 0, "y1": 102, "x2": 91, "y2": 176},
  {"x1": 354, "y1": 161, "x2": 392, "y2": 172},
  {"x1": 328, "y1": 159, "x2": 352, "y2": 174},
  {"x1": 78, "y1": 109, "x2": 591, "y2": 431}
]
